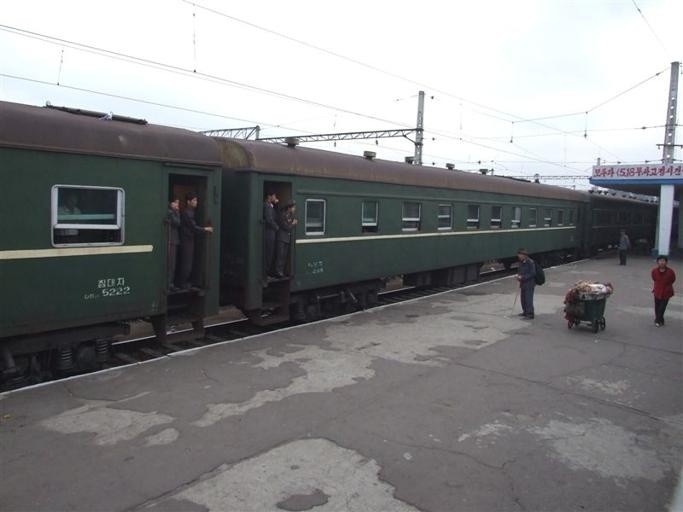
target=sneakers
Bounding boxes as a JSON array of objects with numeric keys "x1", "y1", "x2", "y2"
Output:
[
  {"x1": 518, "y1": 313, "x2": 534, "y2": 321},
  {"x1": 655, "y1": 321, "x2": 664, "y2": 328},
  {"x1": 169, "y1": 281, "x2": 193, "y2": 292},
  {"x1": 268, "y1": 270, "x2": 285, "y2": 278}
]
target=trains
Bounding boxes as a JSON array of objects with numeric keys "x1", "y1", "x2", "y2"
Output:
[{"x1": 0, "y1": 102, "x2": 679, "y2": 380}]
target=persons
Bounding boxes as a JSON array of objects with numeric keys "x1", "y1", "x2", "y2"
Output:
[
  {"x1": 167, "y1": 194, "x2": 181, "y2": 292},
  {"x1": 276, "y1": 199, "x2": 299, "y2": 278},
  {"x1": 263, "y1": 188, "x2": 278, "y2": 279},
  {"x1": 512, "y1": 247, "x2": 536, "y2": 319},
  {"x1": 180, "y1": 192, "x2": 214, "y2": 291},
  {"x1": 56, "y1": 195, "x2": 80, "y2": 238},
  {"x1": 617, "y1": 228, "x2": 630, "y2": 265},
  {"x1": 649, "y1": 255, "x2": 676, "y2": 328}
]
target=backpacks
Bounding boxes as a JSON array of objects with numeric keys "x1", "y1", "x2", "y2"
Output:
[{"x1": 528, "y1": 259, "x2": 545, "y2": 286}]
